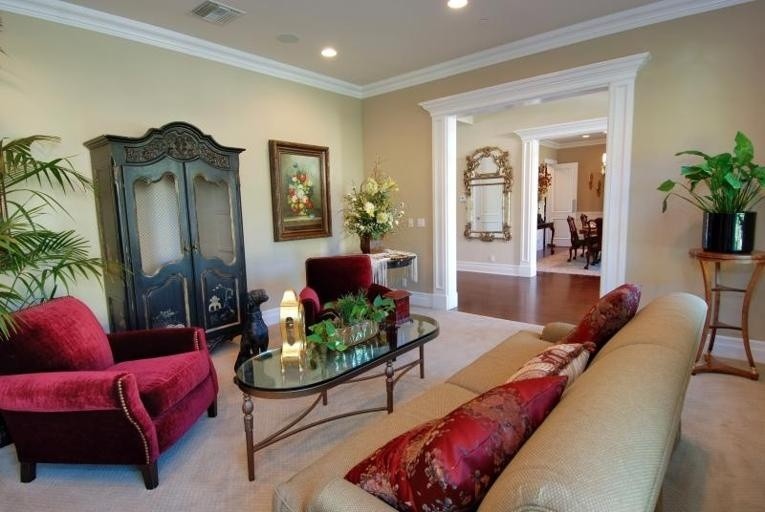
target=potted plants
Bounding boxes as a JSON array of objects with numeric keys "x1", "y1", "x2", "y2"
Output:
[
  {"x1": 655, "y1": 129, "x2": 765, "y2": 254},
  {"x1": 305, "y1": 289, "x2": 400, "y2": 362}
]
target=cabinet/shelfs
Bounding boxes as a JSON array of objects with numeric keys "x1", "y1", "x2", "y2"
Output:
[{"x1": 81, "y1": 122, "x2": 248, "y2": 357}]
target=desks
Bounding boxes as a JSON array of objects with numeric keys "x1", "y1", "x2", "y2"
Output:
[
  {"x1": 687, "y1": 246, "x2": 765, "y2": 381},
  {"x1": 350, "y1": 248, "x2": 419, "y2": 293},
  {"x1": 537, "y1": 222, "x2": 555, "y2": 257}
]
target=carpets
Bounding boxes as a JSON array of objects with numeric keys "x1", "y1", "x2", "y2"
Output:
[{"x1": 536, "y1": 249, "x2": 600, "y2": 278}]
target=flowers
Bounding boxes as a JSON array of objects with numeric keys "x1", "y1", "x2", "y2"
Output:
[
  {"x1": 538, "y1": 163, "x2": 552, "y2": 202},
  {"x1": 341, "y1": 153, "x2": 407, "y2": 239}
]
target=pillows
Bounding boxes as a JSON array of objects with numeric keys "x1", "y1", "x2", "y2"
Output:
[
  {"x1": 555, "y1": 283, "x2": 644, "y2": 349},
  {"x1": 503, "y1": 340, "x2": 602, "y2": 388},
  {"x1": 343, "y1": 374, "x2": 571, "y2": 511}
]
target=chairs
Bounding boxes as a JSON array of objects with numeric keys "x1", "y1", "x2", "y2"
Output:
[
  {"x1": 567, "y1": 215, "x2": 590, "y2": 263},
  {"x1": 579, "y1": 213, "x2": 595, "y2": 257},
  {"x1": 583, "y1": 216, "x2": 602, "y2": 270}
]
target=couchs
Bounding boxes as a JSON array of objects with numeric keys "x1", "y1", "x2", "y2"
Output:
[
  {"x1": 298, "y1": 255, "x2": 413, "y2": 336},
  {"x1": 0, "y1": 294, "x2": 220, "y2": 489},
  {"x1": 273, "y1": 290, "x2": 711, "y2": 510}
]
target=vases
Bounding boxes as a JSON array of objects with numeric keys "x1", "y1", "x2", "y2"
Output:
[{"x1": 357, "y1": 234, "x2": 374, "y2": 254}]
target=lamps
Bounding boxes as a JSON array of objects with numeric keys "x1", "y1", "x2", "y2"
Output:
[{"x1": 599, "y1": 133, "x2": 606, "y2": 175}]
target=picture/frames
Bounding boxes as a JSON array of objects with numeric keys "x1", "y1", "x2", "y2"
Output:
[
  {"x1": 268, "y1": 140, "x2": 336, "y2": 242},
  {"x1": 468, "y1": 178, "x2": 511, "y2": 240}
]
